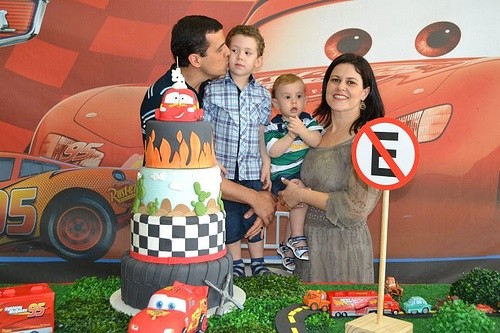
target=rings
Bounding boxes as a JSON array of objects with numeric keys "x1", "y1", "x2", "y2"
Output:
[{"x1": 261, "y1": 226, "x2": 263, "y2": 229}]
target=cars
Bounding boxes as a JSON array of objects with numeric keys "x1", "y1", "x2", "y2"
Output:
[
  {"x1": 127, "y1": 280, "x2": 209, "y2": 333},
  {"x1": 401, "y1": 296, "x2": 431, "y2": 314},
  {"x1": 302, "y1": 289, "x2": 330, "y2": 311}
]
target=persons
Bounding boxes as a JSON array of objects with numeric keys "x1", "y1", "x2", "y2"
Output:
[
  {"x1": 202, "y1": 25, "x2": 273, "y2": 280},
  {"x1": 264, "y1": 74, "x2": 327, "y2": 272},
  {"x1": 277, "y1": 52, "x2": 386, "y2": 284},
  {"x1": 140, "y1": 16, "x2": 279, "y2": 241}
]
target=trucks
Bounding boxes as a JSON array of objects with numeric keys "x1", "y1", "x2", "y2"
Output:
[
  {"x1": 0, "y1": 282, "x2": 55, "y2": 333},
  {"x1": 326, "y1": 290, "x2": 400, "y2": 317}
]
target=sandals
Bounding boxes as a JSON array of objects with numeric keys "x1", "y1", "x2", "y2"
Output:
[
  {"x1": 233, "y1": 263, "x2": 246, "y2": 277},
  {"x1": 286, "y1": 235, "x2": 309, "y2": 259},
  {"x1": 251, "y1": 261, "x2": 272, "y2": 276},
  {"x1": 277, "y1": 243, "x2": 296, "y2": 270}
]
